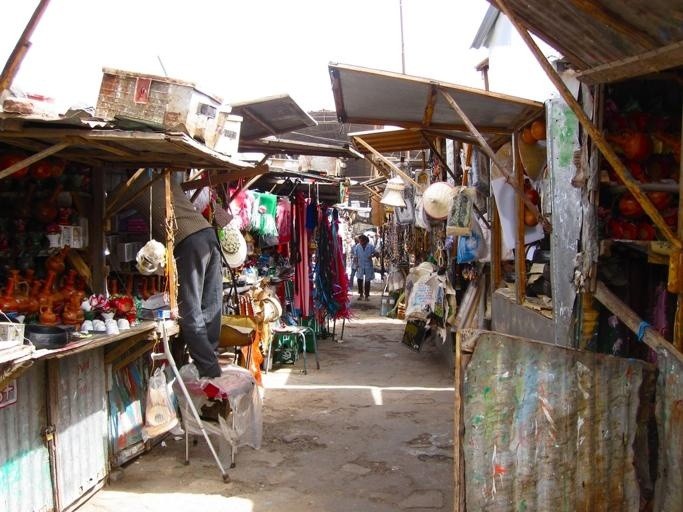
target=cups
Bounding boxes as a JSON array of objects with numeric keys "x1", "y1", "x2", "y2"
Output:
[{"x1": 82, "y1": 319, "x2": 129, "y2": 334}]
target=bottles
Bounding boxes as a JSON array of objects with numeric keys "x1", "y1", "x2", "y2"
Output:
[{"x1": 1, "y1": 268, "x2": 84, "y2": 326}]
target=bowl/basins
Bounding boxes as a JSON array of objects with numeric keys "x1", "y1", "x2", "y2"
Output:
[{"x1": 24, "y1": 325, "x2": 67, "y2": 349}]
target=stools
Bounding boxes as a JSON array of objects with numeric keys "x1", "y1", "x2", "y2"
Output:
[
  {"x1": 266, "y1": 325, "x2": 320, "y2": 375},
  {"x1": 172, "y1": 362, "x2": 255, "y2": 468}
]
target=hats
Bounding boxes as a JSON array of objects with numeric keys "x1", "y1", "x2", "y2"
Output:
[{"x1": 220, "y1": 225, "x2": 248, "y2": 268}]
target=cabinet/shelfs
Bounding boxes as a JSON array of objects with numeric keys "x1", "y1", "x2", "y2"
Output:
[{"x1": 1, "y1": 300, "x2": 202, "y2": 512}]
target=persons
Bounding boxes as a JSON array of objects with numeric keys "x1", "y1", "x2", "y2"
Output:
[
  {"x1": 350, "y1": 234, "x2": 381, "y2": 303},
  {"x1": 345, "y1": 235, "x2": 359, "y2": 291},
  {"x1": 105, "y1": 169, "x2": 228, "y2": 378}
]
